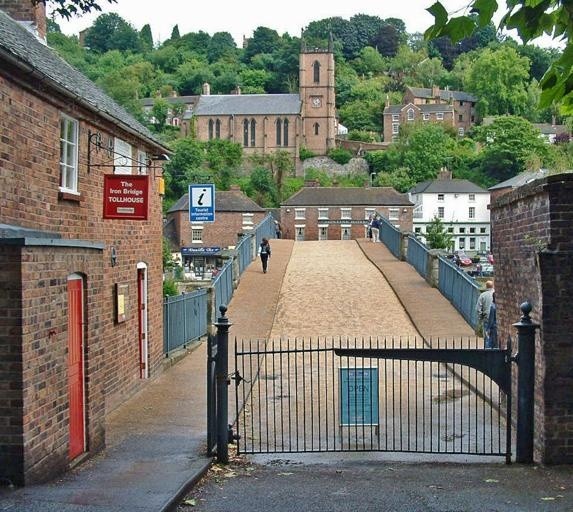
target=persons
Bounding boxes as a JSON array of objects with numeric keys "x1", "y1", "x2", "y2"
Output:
[
  {"x1": 259, "y1": 238, "x2": 272, "y2": 274},
  {"x1": 476, "y1": 280, "x2": 495, "y2": 349},
  {"x1": 368, "y1": 213, "x2": 376, "y2": 238},
  {"x1": 452, "y1": 246, "x2": 493, "y2": 277},
  {"x1": 486, "y1": 291, "x2": 498, "y2": 349},
  {"x1": 371, "y1": 215, "x2": 382, "y2": 243}
]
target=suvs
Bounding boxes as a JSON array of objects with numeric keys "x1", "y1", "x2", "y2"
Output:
[
  {"x1": 453, "y1": 250, "x2": 465, "y2": 257},
  {"x1": 456, "y1": 256, "x2": 471, "y2": 266}
]
